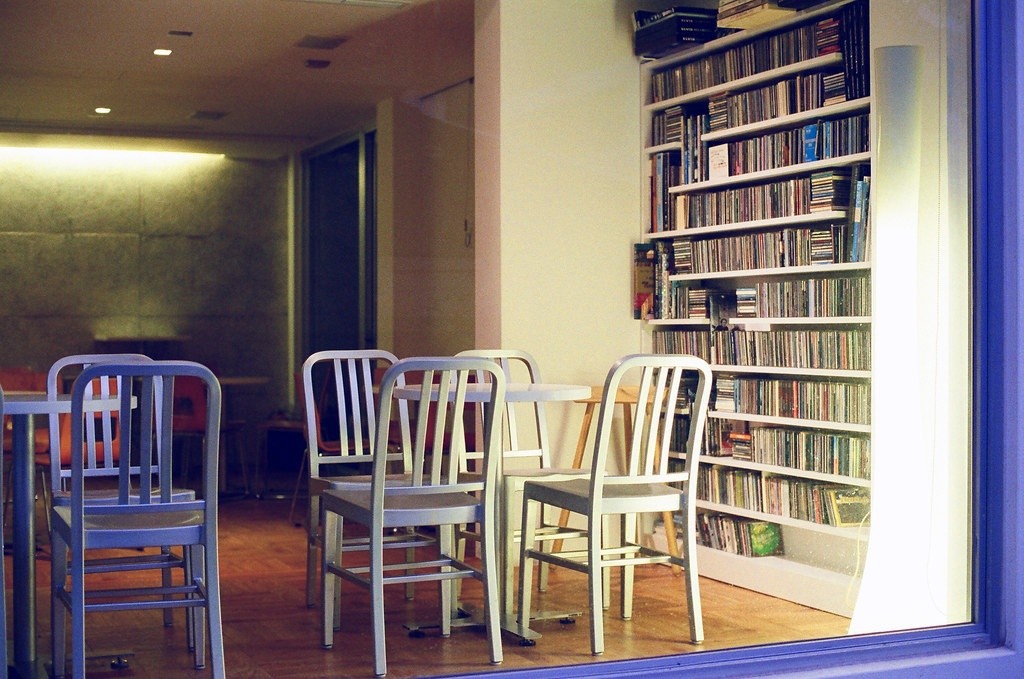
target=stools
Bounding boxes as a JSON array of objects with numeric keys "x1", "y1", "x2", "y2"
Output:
[{"x1": 548, "y1": 386, "x2": 682, "y2": 578}]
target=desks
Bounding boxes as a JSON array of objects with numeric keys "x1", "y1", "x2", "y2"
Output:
[
  {"x1": 201, "y1": 374, "x2": 270, "y2": 502},
  {"x1": 393, "y1": 382, "x2": 591, "y2": 647},
  {"x1": 0, "y1": 395, "x2": 137, "y2": 679}
]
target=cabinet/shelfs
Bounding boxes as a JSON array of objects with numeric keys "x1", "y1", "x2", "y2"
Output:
[{"x1": 642, "y1": 0, "x2": 950, "y2": 618}]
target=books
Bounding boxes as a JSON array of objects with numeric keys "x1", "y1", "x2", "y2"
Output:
[{"x1": 630, "y1": 0, "x2": 872, "y2": 557}]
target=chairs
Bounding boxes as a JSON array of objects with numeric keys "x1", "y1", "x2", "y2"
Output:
[
  {"x1": 251, "y1": 349, "x2": 712, "y2": 679},
  {"x1": 0, "y1": 353, "x2": 226, "y2": 679}
]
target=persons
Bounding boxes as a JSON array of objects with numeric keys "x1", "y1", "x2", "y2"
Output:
[{"x1": 715, "y1": 318, "x2": 728, "y2": 331}]
